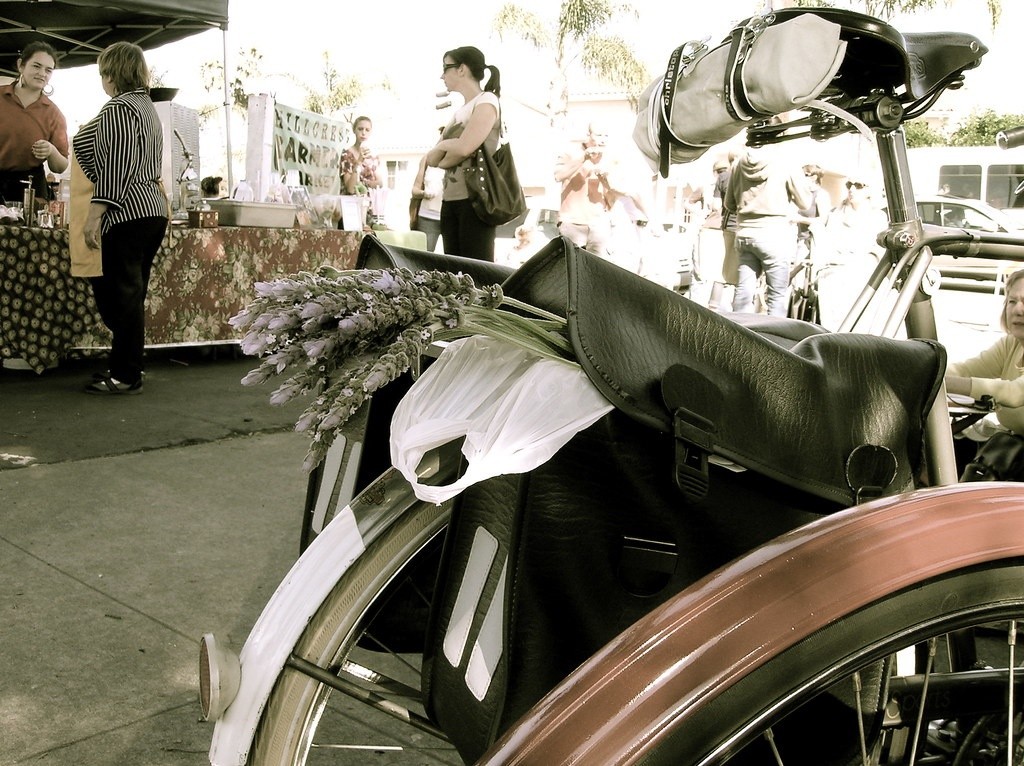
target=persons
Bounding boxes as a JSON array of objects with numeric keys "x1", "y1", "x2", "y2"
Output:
[
  {"x1": 334, "y1": 116, "x2": 391, "y2": 231},
  {"x1": 201, "y1": 177, "x2": 222, "y2": 197},
  {"x1": 508, "y1": 226, "x2": 534, "y2": 267},
  {"x1": 687, "y1": 117, "x2": 888, "y2": 319},
  {"x1": 413, "y1": 127, "x2": 445, "y2": 253},
  {"x1": 0, "y1": 42, "x2": 69, "y2": 210},
  {"x1": 946, "y1": 269, "x2": 1024, "y2": 479},
  {"x1": 69, "y1": 42, "x2": 172, "y2": 394},
  {"x1": 428, "y1": 46, "x2": 500, "y2": 263},
  {"x1": 556, "y1": 121, "x2": 626, "y2": 259}
]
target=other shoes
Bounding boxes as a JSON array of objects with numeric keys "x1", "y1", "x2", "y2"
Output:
[
  {"x1": 92, "y1": 370, "x2": 111, "y2": 381},
  {"x1": 86, "y1": 377, "x2": 145, "y2": 395}
]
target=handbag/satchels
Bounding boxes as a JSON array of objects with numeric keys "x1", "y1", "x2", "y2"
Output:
[
  {"x1": 423, "y1": 236, "x2": 948, "y2": 766},
  {"x1": 692, "y1": 210, "x2": 738, "y2": 286},
  {"x1": 463, "y1": 90, "x2": 527, "y2": 226},
  {"x1": 339, "y1": 185, "x2": 370, "y2": 231},
  {"x1": 389, "y1": 335, "x2": 616, "y2": 507},
  {"x1": 409, "y1": 197, "x2": 422, "y2": 230},
  {"x1": 299, "y1": 235, "x2": 513, "y2": 653},
  {"x1": 633, "y1": 13, "x2": 872, "y2": 179}
]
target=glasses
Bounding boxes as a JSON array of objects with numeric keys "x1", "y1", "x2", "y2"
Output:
[
  {"x1": 847, "y1": 181, "x2": 866, "y2": 189},
  {"x1": 443, "y1": 64, "x2": 460, "y2": 74},
  {"x1": 805, "y1": 173, "x2": 812, "y2": 177}
]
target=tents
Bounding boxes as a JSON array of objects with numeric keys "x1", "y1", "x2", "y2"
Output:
[{"x1": 0, "y1": 0, "x2": 233, "y2": 194}]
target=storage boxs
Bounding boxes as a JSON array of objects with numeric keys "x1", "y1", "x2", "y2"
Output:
[{"x1": 207, "y1": 199, "x2": 298, "y2": 227}]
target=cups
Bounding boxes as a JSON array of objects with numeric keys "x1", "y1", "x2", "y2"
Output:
[
  {"x1": 319, "y1": 198, "x2": 338, "y2": 229},
  {"x1": 5, "y1": 201, "x2": 23, "y2": 211}
]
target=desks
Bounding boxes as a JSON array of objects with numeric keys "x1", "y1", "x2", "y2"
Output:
[{"x1": 0, "y1": 218, "x2": 365, "y2": 373}]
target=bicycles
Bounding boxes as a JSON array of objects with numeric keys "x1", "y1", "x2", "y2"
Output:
[{"x1": 200, "y1": 5, "x2": 1024, "y2": 766}]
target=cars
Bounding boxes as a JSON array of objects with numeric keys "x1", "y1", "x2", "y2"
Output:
[{"x1": 882, "y1": 196, "x2": 1024, "y2": 290}]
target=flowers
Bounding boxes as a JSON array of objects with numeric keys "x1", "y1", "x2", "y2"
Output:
[{"x1": 229, "y1": 265, "x2": 585, "y2": 472}]
